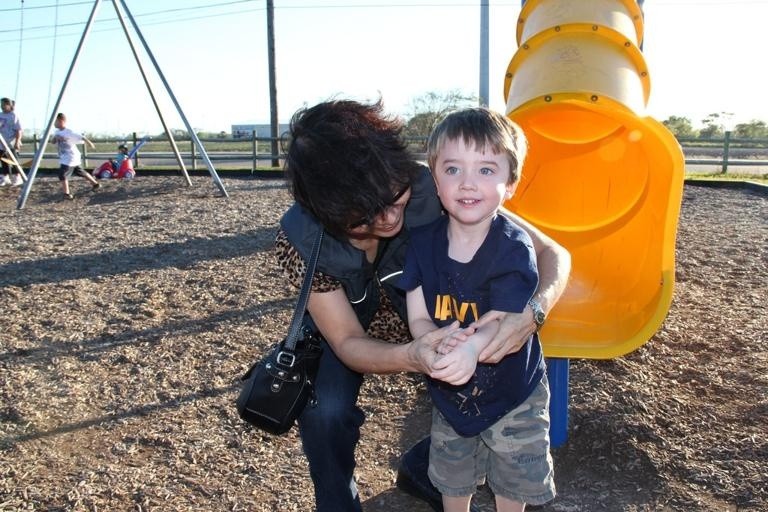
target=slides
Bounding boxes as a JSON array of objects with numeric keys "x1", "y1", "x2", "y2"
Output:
[{"x1": 502, "y1": 0, "x2": 685, "y2": 360}]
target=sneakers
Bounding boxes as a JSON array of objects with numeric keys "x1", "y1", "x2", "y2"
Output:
[
  {"x1": 0, "y1": 174, "x2": 11, "y2": 187},
  {"x1": 58, "y1": 192, "x2": 74, "y2": 202},
  {"x1": 86, "y1": 183, "x2": 102, "y2": 194},
  {"x1": 12, "y1": 174, "x2": 24, "y2": 186},
  {"x1": 394, "y1": 452, "x2": 482, "y2": 512}
]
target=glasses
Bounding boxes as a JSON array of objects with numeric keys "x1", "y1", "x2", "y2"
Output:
[{"x1": 347, "y1": 182, "x2": 411, "y2": 229}]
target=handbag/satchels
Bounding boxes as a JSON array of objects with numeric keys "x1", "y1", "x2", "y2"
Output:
[{"x1": 235, "y1": 325, "x2": 325, "y2": 436}]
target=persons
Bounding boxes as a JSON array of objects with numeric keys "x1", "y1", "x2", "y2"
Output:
[
  {"x1": 394, "y1": 107, "x2": 558, "y2": 512},
  {"x1": 272, "y1": 88, "x2": 576, "y2": 512},
  {"x1": 0, "y1": 97, "x2": 26, "y2": 188},
  {"x1": 109, "y1": 143, "x2": 130, "y2": 171},
  {"x1": 47, "y1": 112, "x2": 104, "y2": 202}
]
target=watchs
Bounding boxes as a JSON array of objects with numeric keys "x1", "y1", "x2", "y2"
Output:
[{"x1": 528, "y1": 297, "x2": 547, "y2": 334}]
